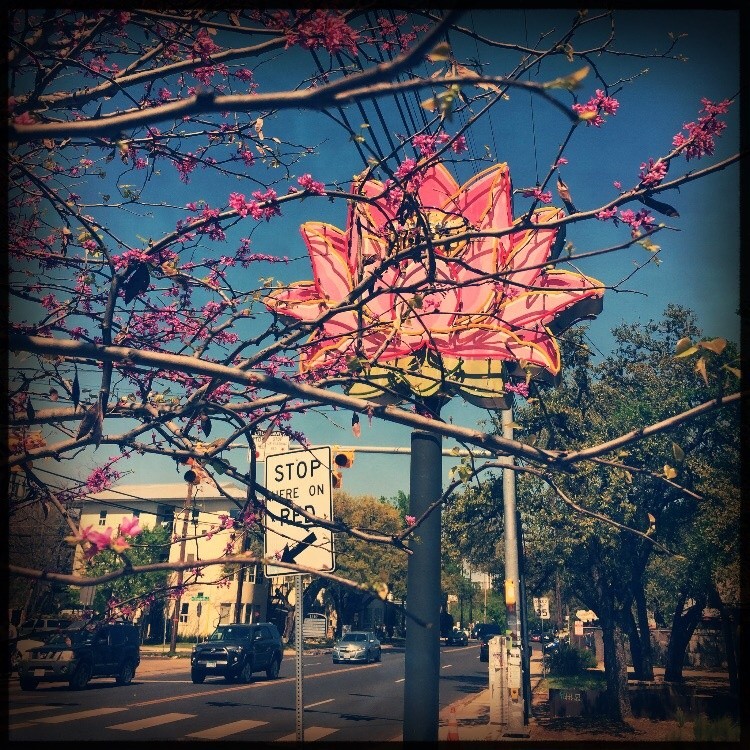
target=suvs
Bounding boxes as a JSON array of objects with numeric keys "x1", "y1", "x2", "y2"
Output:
[
  {"x1": 332, "y1": 630, "x2": 381, "y2": 664},
  {"x1": 541, "y1": 632, "x2": 554, "y2": 643},
  {"x1": 190, "y1": 621, "x2": 284, "y2": 684},
  {"x1": 17, "y1": 620, "x2": 142, "y2": 691},
  {"x1": 478, "y1": 634, "x2": 500, "y2": 662}
]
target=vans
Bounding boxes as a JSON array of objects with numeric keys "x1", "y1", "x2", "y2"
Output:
[
  {"x1": 470, "y1": 623, "x2": 501, "y2": 640},
  {"x1": 17, "y1": 618, "x2": 72, "y2": 639}
]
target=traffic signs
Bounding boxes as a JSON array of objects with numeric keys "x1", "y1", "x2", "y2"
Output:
[{"x1": 263, "y1": 445, "x2": 336, "y2": 579}]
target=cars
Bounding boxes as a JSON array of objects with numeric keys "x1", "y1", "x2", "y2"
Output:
[
  {"x1": 445, "y1": 633, "x2": 469, "y2": 647},
  {"x1": 531, "y1": 634, "x2": 541, "y2": 642}
]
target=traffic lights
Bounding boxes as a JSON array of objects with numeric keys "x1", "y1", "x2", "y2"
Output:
[
  {"x1": 179, "y1": 447, "x2": 202, "y2": 485},
  {"x1": 330, "y1": 449, "x2": 352, "y2": 489}
]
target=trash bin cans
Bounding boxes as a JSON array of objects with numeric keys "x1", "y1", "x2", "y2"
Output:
[
  {"x1": 549, "y1": 688, "x2": 600, "y2": 722},
  {"x1": 628, "y1": 679, "x2": 686, "y2": 720}
]
